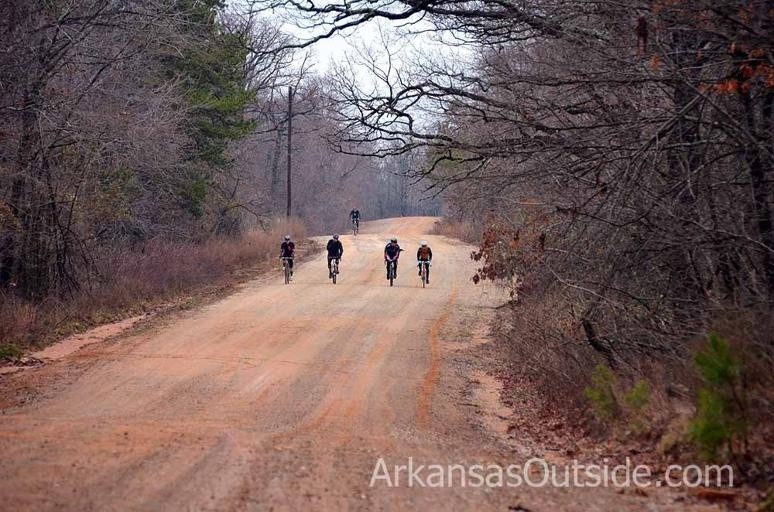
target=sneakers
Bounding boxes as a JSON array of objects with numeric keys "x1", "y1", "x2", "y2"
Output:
[
  {"x1": 418, "y1": 271, "x2": 422, "y2": 275},
  {"x1": 337, "y1": 271, "x2": 339, "y2": 274},
  {"x1": 328, "y1": 273, "x2": 333, "y2": 279},
  {"x1": 394, "y1": 274, "x2": 397, "y2": 278},
  {"x1": 426, "y1": 279, "x2": 429, "y2": 284},
  {"x1": 386, "y1": 275, "x2": 390, "y2": 280}
]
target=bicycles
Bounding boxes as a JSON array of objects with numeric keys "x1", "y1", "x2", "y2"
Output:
[
  {"x1": 351, "y1": 218, "x2": 359, "y2": 236},
  {"x1": 280, "y1": 257, "x2": 292, "y2": 284},
  {"x1": 328, "y1": 257, "x2": 339, "y2": 283},
  {"x1": 385, "y1": 256, "x2": 397, "y2": 286},
  {"x1": 417, "y1": 259, "x2": 432, "y2": 288}
]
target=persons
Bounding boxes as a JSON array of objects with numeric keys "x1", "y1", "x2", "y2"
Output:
[
  {"x1": 348, "y1": 207, "x2": 361, "y2": 231},
  {"x1": 383, "y1": 237, "x2": 401, "y2": 280},
  {"x1": 279, "y1": 235, "x2": 295, "y2": 282},
  {"x1": 417, "y1": 241, "x2": 433, "y2": 284},
  {"x1": 326, "y1": 234, "x2": 343, "y2": 279}
]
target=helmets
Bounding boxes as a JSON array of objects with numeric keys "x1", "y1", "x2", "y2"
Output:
[
  {"x1": 421, "y1": 240, "x2": 428, "y2": 248},
  {"x1": 284, "y1": 235, "x2": 291, "y2": 240},
  {"x1": 390, "y1": 238, "x2": 397, "y2": 243},
  {"x1": 332, "y1": 234, "x2": 339, "y2": 239}
]
